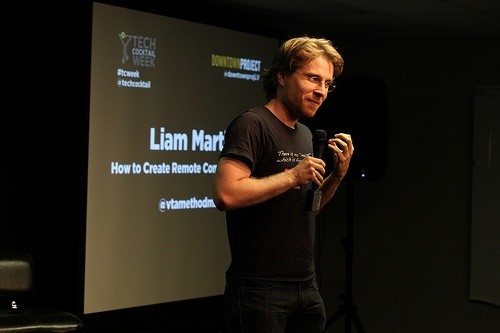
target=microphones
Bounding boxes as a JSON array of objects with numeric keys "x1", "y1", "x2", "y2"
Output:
[{"x1": 312, "y1": 130, "x2": 327, "y2": 189}]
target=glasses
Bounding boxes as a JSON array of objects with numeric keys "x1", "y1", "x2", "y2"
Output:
[{"x1": 296, "y1": 71, "x2": 336, "y2": 93}]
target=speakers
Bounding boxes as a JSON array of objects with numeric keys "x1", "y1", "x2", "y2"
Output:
[{"x1": 329, "y1": 74, "x2": 393, "y2": 187}]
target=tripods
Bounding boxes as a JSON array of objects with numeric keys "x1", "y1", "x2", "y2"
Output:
[{"x1": 325, "y1": 187, "x2": 370, "y2": 333}]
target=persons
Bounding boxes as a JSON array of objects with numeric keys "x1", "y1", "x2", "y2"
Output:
[{"x1": 212, "y1": 37, "x2": 354, "y2": 333}]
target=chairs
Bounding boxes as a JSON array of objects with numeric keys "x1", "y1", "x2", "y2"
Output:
[{"x1": 0, "y1": 260, "x2": 82, "y2": 333}]
